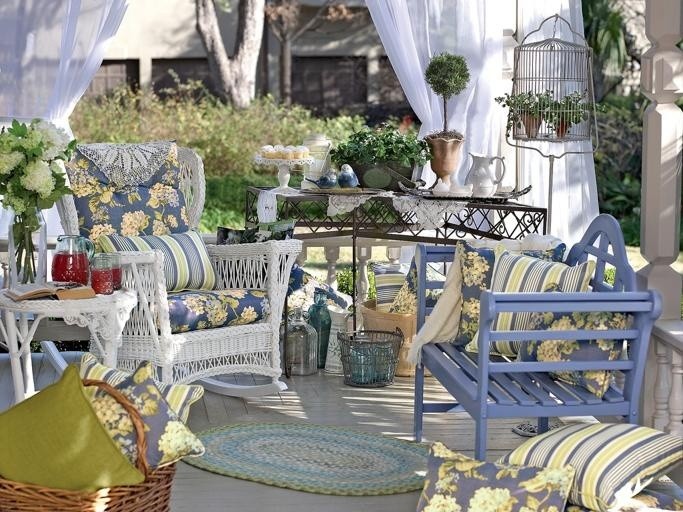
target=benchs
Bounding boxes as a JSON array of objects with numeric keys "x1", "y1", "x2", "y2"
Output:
[{"x1": 414, "y1": 213, "x2": 663, "y2": 461}]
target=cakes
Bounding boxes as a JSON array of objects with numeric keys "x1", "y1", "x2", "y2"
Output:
[{"x1": 259, "y1": 145, "x2": 310, "y2": 160}]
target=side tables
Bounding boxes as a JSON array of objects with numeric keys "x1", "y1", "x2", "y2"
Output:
[{"x1": 1, "y1": 282, "x2": 137, "y2": 404}]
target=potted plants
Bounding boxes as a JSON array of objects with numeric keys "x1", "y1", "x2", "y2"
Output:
[
  {"x1": 329, "y1": 48, "x2": 471, "y2": 192},
  {"x1": 495, "y1": 88, "x2": 608, "y2": 138}
]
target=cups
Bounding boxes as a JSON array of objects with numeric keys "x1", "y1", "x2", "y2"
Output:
[
  {"x1": 50, "y1": 235, "x2": 93, "y2": 286},
  {"x1": 463, "y1": 151, "x2": 506, "y2": 199},
  {"x1": 90, "y1": 253, "x2": 120, "y2": 295}
]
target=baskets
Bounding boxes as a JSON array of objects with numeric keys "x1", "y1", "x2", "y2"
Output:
[
  {"x1": 0, "y1": 378, "x2": 176, "y2": 512},
  {"x1": 337, "y1": 328, "x2": 404, "y2": 387}
]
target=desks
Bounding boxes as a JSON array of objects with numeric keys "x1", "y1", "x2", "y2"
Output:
[{"x1": 244, "y1": 186, "x2": 547, "y2": 337}]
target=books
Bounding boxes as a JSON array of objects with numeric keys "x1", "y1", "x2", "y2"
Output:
[{"x1": 2, "y1": 282, "x2": 95, "y2": 302}]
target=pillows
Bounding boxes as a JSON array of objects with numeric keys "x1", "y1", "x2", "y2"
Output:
[
  {"x1": 217, "y1": 218, "x2": 352, "y2": 309},
  {"x1": 65, "y1": 139, "x2": 219, "y2": 293},
  {"x1": 416, "y1": 423, "x2": 683, "y2": 512},
  {"x1": 0, "y1": 352, "x2": 205, "y2": 489},
  {"x1": 456, "y1": 239, "x2": 634, "y2": 398}
]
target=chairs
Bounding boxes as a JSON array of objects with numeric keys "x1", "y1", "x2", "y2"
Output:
[{"x1": 52, "y1": 151, "x2": 304, "y2": 398}]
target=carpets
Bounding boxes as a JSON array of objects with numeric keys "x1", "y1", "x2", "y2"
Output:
[{"x1": 181, "y1": 419, "x2": 430, "y2": 497}]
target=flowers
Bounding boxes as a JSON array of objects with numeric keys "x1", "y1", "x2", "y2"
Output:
[{"x1": 0, "y1": 117, "x2": 78, "y2": 285}]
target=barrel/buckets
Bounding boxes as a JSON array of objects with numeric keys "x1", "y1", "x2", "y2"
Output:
[{"x1": 359, "y1": 302, "x2": 434, "y2": 376}]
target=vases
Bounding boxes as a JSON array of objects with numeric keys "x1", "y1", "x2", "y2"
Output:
[{"x1": 8, "y1": 207, "x2": 47, "y2": 293}]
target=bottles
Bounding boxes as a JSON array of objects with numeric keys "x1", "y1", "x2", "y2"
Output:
[
  {"x1": 301, "y1": 135, "x2": 331, "y2": 189},
  {"x1": 274, "y1": 306, "x2": 318, "y2": 375}
]
